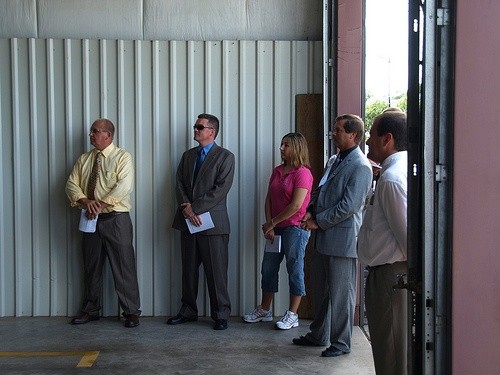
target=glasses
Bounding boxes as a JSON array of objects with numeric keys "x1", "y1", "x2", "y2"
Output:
[
  {"x1": 89, "y1": 129, "x2": 110, "y2": 134},
  {"x1": 193, "y1": 124, "x2": 214, "y2": 132}
]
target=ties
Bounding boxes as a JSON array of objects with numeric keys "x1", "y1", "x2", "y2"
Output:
[
  {"x1": 193, "y1": 148, "x2": 204, "y2": 188},
  {"x1": 87, "y1": 152, "x2": 102, "y2": 202},
  {"x1": 327, "y1": 152, "x2": 341, "y2": 182}
]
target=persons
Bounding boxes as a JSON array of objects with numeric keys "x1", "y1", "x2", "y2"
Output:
[
  {"x1": 65, "y1": 119, "x2": 142, "y2": 327},
  {"x1": 292, "y1": 115, "x2": 373, "y2": 356},
  {"x1": 370, "y1": 107, "x2": 403, "y2": 181},
  {"x1": 167, "y1": 113, "x2": 235, "y2": 329},
  {"x1": 356, "y1": 111, "x2": 408, "y2": 375},
  {"x1": 242, "y1": 132, "x2": 313, "y2": 329}
]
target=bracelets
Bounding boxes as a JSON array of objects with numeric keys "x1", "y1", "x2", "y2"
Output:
[{"x1": 272, "y1": 219, "x2": 276, "y2": 227}]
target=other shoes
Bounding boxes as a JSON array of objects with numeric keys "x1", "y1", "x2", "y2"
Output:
[
  {"x1": 292, "y1": 335, "x2": 328, "y2": 347},
  {"x1": 321, "y1": 346, "x2": 351, "y2": 358}
]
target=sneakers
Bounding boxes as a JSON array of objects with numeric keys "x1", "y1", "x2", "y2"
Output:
[
  {"x1": 242, "y1": 305, "x2": 274, "y2": 322},
  {"x1": 275, "y1": 310, "x2": 300, "y2": 330}
]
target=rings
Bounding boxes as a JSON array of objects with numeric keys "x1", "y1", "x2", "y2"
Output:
[{"x1": 193, "y1": 219, "x2": 194, "y2": 220}]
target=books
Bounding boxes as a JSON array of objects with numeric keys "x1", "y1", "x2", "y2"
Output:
[
  {"x1": 185, "y1": 211, "x2": 215, "y2": 234},
  {"x1": 79, "y1": 210, "x2": 98, "y2": 232},
  {"x1": 266, "y1": 236, "x2": 281, "y2": 252}
]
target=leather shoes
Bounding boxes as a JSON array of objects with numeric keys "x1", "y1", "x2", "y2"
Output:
[
  {"x1": 213, "y1": 319, "x2": 229, "y2": 330},
  {"x1": 167, "y1": 315, "x2": 199, "y2": 324},
  {"x1": 71, "y1": 313, "x2": 101, "y2": 325},
  {"x1": 125, "y1": 314, "x2": 141, "y2": 327}
]
